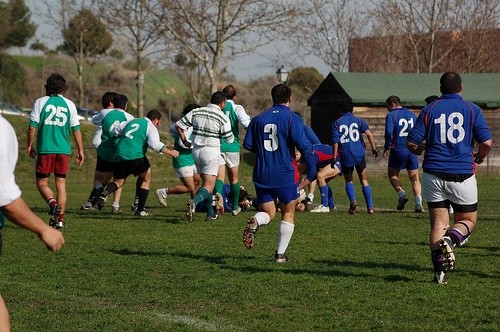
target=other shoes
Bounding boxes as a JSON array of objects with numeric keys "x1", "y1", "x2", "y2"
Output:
[
  {"x1": 80, "y1": 204, "x2": 97, "y2": 211},
  {"x1": 368, "y1": 207, "x2": 375, "y2": 214},
  {"x1": 243, "y1": 216, "x2": 259, "y2": 249},
  {"x1": 275, "y1": 253, "x2": 290, "y2": 263},
  {"x1": 185, "y1": 199, "x2": 195, "y2": 223},
  {"x1": 415, "y1": 204, "x2": 424, "y2": 213},
  {"x1": 131, "y1": 205, "x2": 145, "y2": 211},
  {"x1": 207, "y1": 213, "x2": 220, "y2": 220},
  {"x1": 56, "y1": 221, "x2": 64, "y2": 229},
  {"x1": 232, "y1": 206, "x2": 241, "y2": 217},
  {"x1": 96, "y1": 191, "x2": 108, "y2": 210},
  {"x1": 48, "y1": 204, "x2": 62, "y2": 228},
  {"x1": 330, "y1": 206, "x2": 337, "y2": 211},
  {"x1": 309, "y1": 204, "x2": 330, "y2": 213},
  {"x1": 155, "y1": 188, "x2": 168, "y2": 207},
  {"x1": 397, "y1": 195, "x2": 408, "y2": 211},
  {"x1": 134, "y1": 209, "x2": 153, "y2": 217},
  {"x1": 438, "y1": 236, "x2": 457, "y2": 272},
  {"x1": 348, "y1": 199, "x2": 359, "y2": 215},
  {"x1": 111, "y1": 205, "x2": 123, "y2": 213},
  {"x1": 433, "y1": 270, "x2": 448, "y2": 286},
  {"x1": 213, "y1": 192, "x2": 224, "y2": 215}
]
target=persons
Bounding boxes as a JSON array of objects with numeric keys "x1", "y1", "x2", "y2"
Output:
[
  {"x1": 424, "y1": 95, "x2": 454, "y2": 214},
  {"x1": 175, "y1": 91, "x2": 235, "y2": 223},
  {"x1": 80, "y1": 92, "x2": 180, "y2": 217},
  {"x1": 26, "y1": 72, "x2": 85, "y2": 229},
  {"x1": 406, "y1": 71, "x2": 494, "y2": 283},
  {"x1": 331, "y1": 97, "x2": 379, "y2": 214},
  {"x1": 382, "y1": 96, "x2": 426, "y2": 212},
  {"x1": 154, "y1": 103, "x2": 197, "y2": 206},
  {"x1": 294, "y1": 144, "x2": 343, "y2": 213},
  {"x1": 243, "y1": 84, "x2": 317, "y2": 263},
  {"x1": 0, "y1": 113, "x2": 66, "y2": 332},
  {"x1": 290, "y1": 111, "x2": 322, "y2": 205},
  {"x1": 215, "y1": 84, "x2": 253, "y2": 217},
  {"x1": 193, "y1": 184, "x2": 309, "y2": 212}
]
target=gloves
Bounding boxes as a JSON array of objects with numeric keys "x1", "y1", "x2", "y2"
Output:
[
  {"x1": 372, "y1": 148, "x2": 379, "y2": 158},
  {"x1": 330, "y1": 158, "x2": 337, "y2": 169}
]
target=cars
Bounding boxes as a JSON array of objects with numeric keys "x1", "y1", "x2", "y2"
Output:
[
  {"x1": 0, "y1": 101, "x2": 28, "y2": 117},
  {"x1": 77, "y1": 107, "x2": 99, "y2": 121}
]
